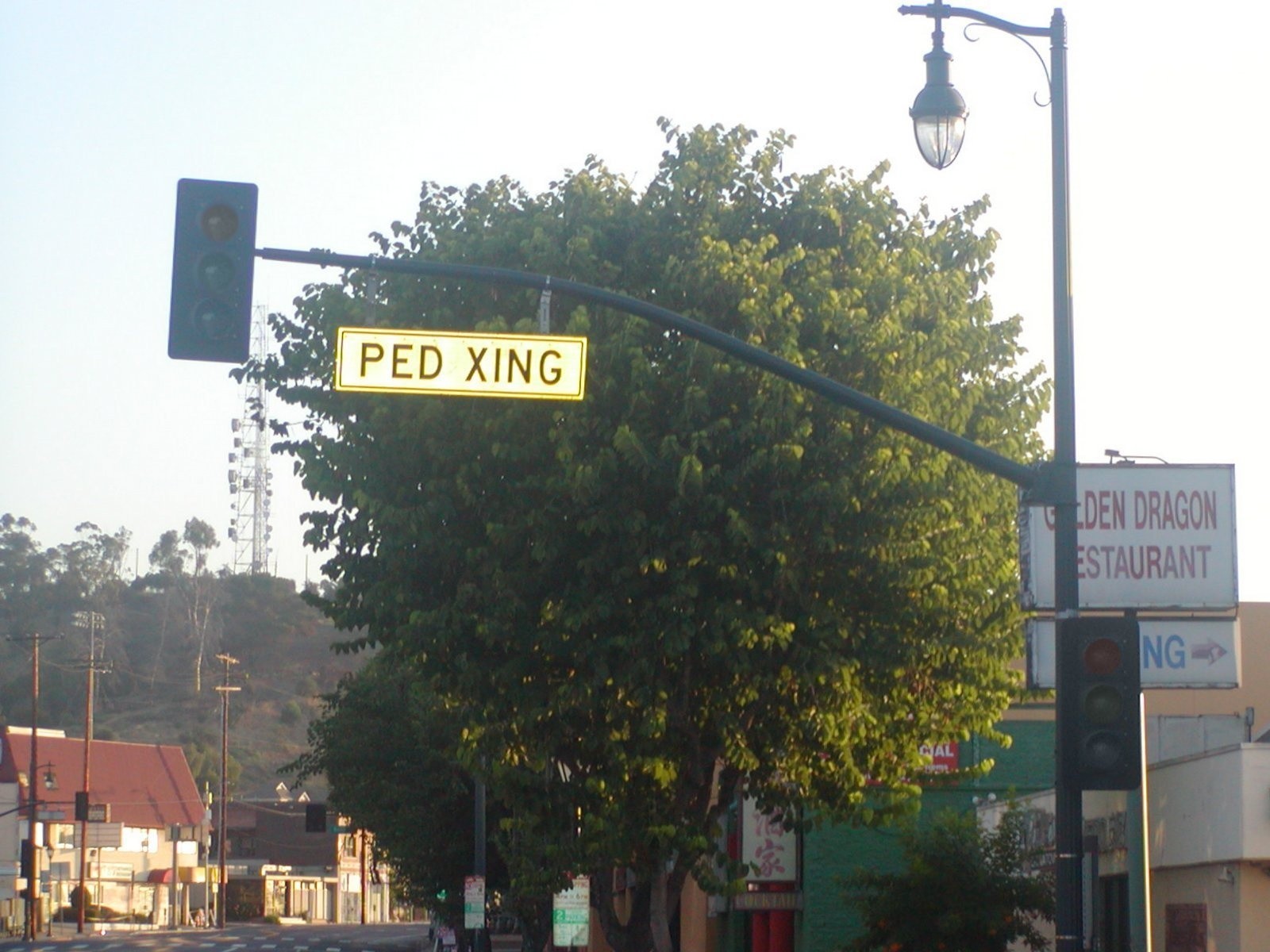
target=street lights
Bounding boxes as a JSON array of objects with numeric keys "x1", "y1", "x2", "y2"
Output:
[
  {"x1": 898, "y1": 2, "x2": 1085, "y2": 952},
  {"x1": 29, "y1": 761, "x2": 57, "y2": 941},
  {"x1": 69, "y1": 608, "x2": 105, "y2": 739},
  {"x1": 45, "y1": 844, "x2": 55, "y2": 937}
]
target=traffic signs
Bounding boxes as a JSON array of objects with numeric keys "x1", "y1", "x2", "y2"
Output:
[
  {"x1": 1025, "y1": 614, "x2": 1242, "y2": 691},
  {"x1": 331, "y1": 825, "x2": 358, "y2": 833}
]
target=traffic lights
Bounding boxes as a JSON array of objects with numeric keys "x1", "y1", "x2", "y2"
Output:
[
  {"x1": 75, "y1": 792, "x2": 88, "y2": 820},
  {"x1": 1059, "y1": 616, "x2": 1142, "y2": 790},
  {"x1": 21, "y1": 838, "x2": 29, "y2": 878},
  {"x1": 168, "y1": 178, "x2": 259, "y2": 362},
  {"x1": 306, "y1": 804, "x2": 326, "y2": 833}
]
